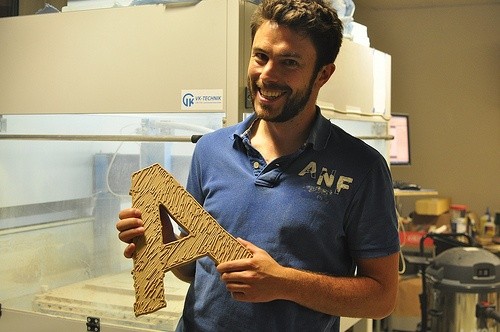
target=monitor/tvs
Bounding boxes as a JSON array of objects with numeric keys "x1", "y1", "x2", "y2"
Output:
[{"x1": 389, "y1": 114, "x2": 410, "y2": 165}]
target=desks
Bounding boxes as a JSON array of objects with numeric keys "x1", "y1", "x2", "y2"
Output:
[{"x1": 394, "y1": 188, "x2": 438, "y2": 211}]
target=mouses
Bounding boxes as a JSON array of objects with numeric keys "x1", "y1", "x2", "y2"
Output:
[{"x1": 399, "y1": 183, "x2": 420, "y2": 190}]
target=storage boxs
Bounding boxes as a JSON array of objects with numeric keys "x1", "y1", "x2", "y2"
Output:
[{"x1": 415, "y1": 198, "x2": 449, "y2": 216}]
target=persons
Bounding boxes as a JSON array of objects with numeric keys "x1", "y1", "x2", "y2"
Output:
[{"x1": 116, "y1": 0, "x2": 400, "y2": 332}]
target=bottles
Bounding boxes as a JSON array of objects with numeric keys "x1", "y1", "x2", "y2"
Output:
[
  {"x1": 483, "y1": 215, "x2": 495, "y2": 237},
  {"x1": 456, "y1": 210, "x2": 467, "y2": 233},
  {"x1": 480, "y1": 207, "x2": 494, "y2": 233}
]
